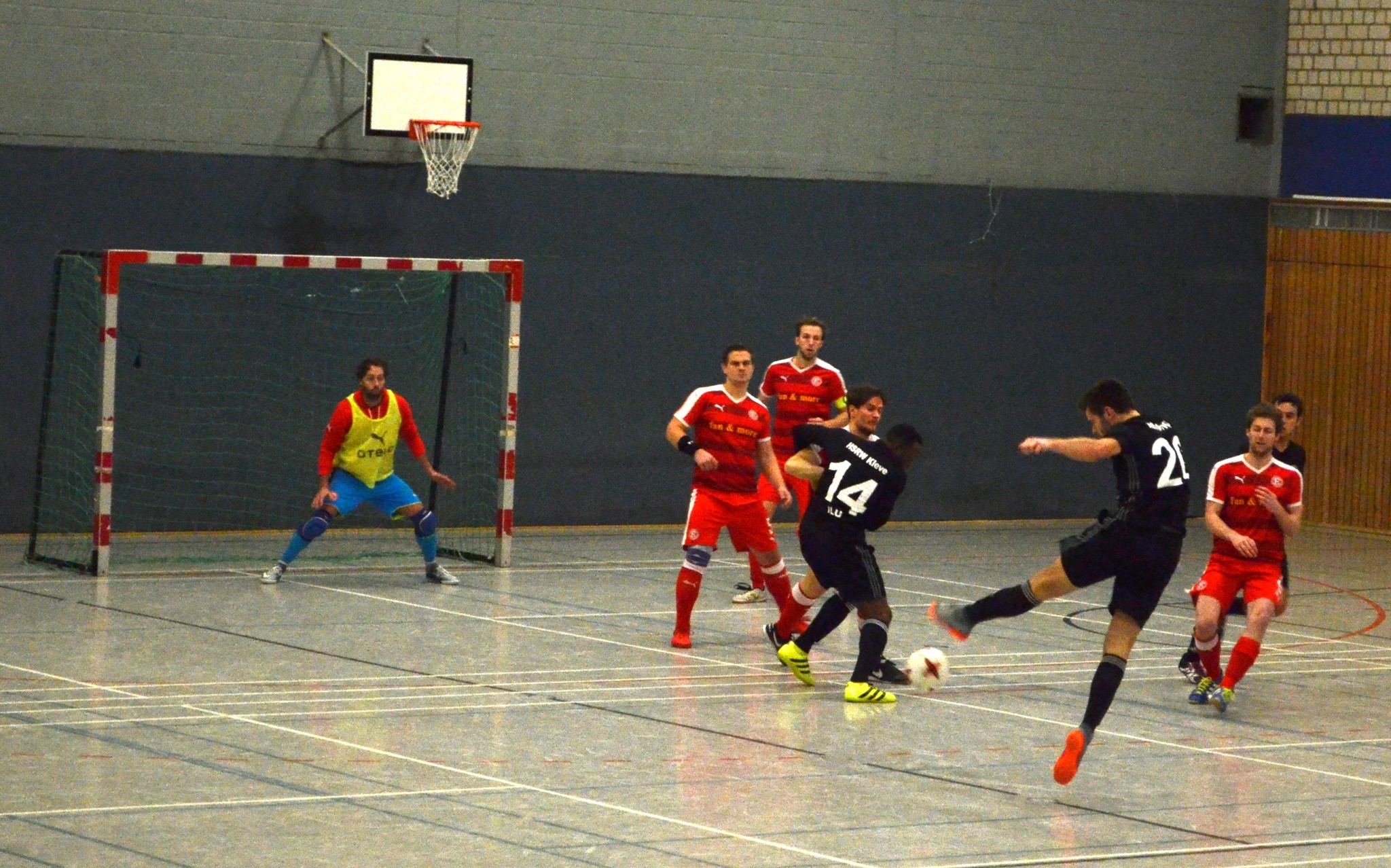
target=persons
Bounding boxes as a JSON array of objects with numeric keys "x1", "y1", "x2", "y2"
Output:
[
  {"x1": 664, "y1": 344, "x2": 817, "y2": 646},
  {"x1": 259, "y1": 358, "x2": 460, "y2": 586},
  {"x1": 1178, "y1": 393, "x2": 1307, "y2": 712},
  {"x1": 750, "y1": 316, "x2": 922, "y2": 703},
  {"x1": 928, "y1": 379, "x2": 1190, "y2": 787}
]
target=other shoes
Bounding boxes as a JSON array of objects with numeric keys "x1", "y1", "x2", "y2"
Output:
[
  {"x1": 1053, "y1": 724, "x2": 1088, "y2": 784},
  {"x1": 924, "y1": 600, "x2": 975, "y2": 641}
]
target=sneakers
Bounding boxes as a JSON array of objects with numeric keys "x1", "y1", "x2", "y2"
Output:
[
  {"x1": 424, "y1": 563, "x2": 459, "y2": 584},
  {"x1": 1178, "y1": 651, "x2": 1208, "y2": 684},
  {"x1": 1208, "y1": 689, "x2": 1235, "y2": 712},
  {"x1": 260, "y1": 565, "x2": 284, "y2": 584},
  {"x1": 671, "y1": 629, "x2": 691, "y2": 649},
  {"x1": 732, "y1": 582, "x2": 766, "y2": 604},
  {"x1": 844, "y1": 679, "x2": 896, "y2": 703},
  {"x1": 793, "y1": 620, "x2": 811, "y2": 635},
  {"x1": 1187, "y1": 668, "x2": 1227, "y2": 704},
  {"x1": 778, "y1": 640, "x2": 816, "y2": 686},
  {"x1": 763, "y1": 623, "x2": 796, "y2": 667},
  {"x1": 867, "y1": 656, "x2": 910, "y2": 685}
]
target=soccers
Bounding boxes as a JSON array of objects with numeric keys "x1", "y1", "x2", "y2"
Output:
[{"x1": 906, "y1": 648, "x2": 949, "y2": 691}]
